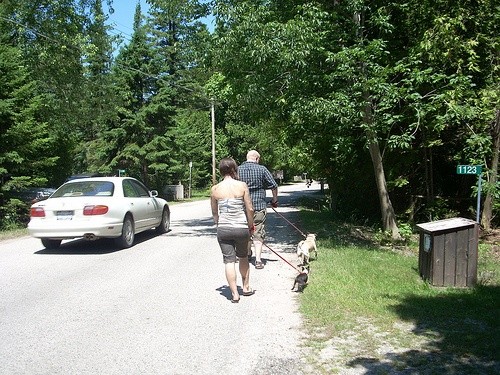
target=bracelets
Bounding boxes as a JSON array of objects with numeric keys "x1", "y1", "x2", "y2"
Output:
[
  {"x1": 271, "y1": 199, "x2": 277, "y2": 204},
  {"x1": 250, "y1": 226, "x2": 255, "y2": 231}
]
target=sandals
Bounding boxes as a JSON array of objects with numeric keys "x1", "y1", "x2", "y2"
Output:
[
  {"x1": 253, "y1": 261, "x2": 264, "y2": 269},
  {"x1": 248, "y1": 250, "x2": 252, "y2": 261}
]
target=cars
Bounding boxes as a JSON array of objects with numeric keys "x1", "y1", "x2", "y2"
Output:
[{"x1": 26, "y1": 176, "x2": 172, "y2": 250}]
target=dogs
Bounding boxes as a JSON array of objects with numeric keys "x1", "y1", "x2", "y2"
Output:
[
  {"x1": 291, "y1": 266, "x2": 310, "y2": 292},
  {"x1": 297, "y1": 232, "x2": 317, "y2": 265}
]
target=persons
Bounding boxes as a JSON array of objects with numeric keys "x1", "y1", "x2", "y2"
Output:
[
  {"x1": 211, "y1": 157, "x2": 256, "y2": 303},
  {"x1": 238, "y1": 150, "x2": 277, "y2": 269}
]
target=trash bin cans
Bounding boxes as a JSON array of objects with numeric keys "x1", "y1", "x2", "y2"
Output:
[{"x1": 416, "y1": 217, "x2": 480, "y2": 288}]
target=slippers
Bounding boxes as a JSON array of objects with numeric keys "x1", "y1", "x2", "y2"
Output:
[
  {"x1": 243, "y1": 287, "x2": 255, "y2": 296},
  {"x1": 231, "y1": 297, "x2": 240, "y2": 303}
]
